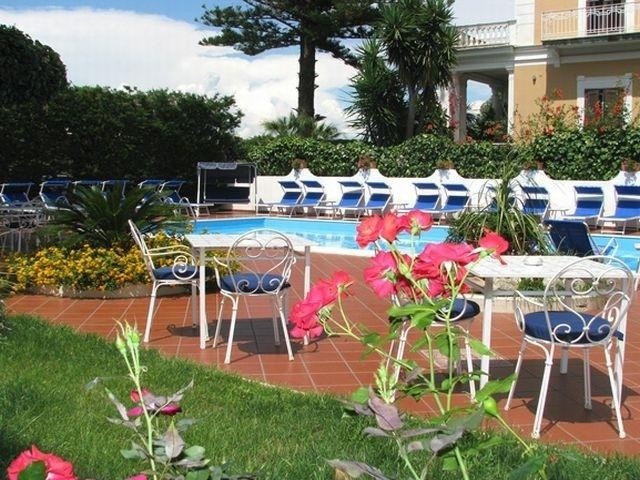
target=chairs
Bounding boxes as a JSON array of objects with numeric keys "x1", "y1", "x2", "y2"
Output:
[
  {"x1": 505, "y1": 255, "x2": 636, "y2": 440},
  {"x1": 1, "y1": 179, "x2": 203, "y2": 257},
  {"x1": 388, "y1": 183, "x2": 441, "y2": 216},
  {"x1": 212, "y1": 229, "x2": 296, "y2": 362},
  {"x1": 255, "y1": 182, "x2": 302, "y2": 216},
  {"x1": 420, "y1": 184, "x2": 471, "y2": 227},
  {"x1": 548, "y1": 185, "x2": 606, "y2": 230},
  {"x1": 544, "y1": 219, "x2": 619, "y2": 262},
  {"x1": 311, "y1": 182, "x2": 365, "y2": 217},
  {"x1": 488, "y1": 187, "x2": 517, "y2": 212},
  {"x1": 339, "y1": 183, "x2": 394, "y2": 221},
  {"x1": 126, "y1": 220, "x2": 215, "y2": 344},
  {"x1": 520, "y1": 185, "x2": 550, "y2": 222},
  {"x1": 375, "y1": 243, "x2": 481, "y2": 400},
  {"x1": 269, "y1": 180, "x2": 326, "y2": 217},
  {"x1": 598, "y1": 185, "x2": 639, "y2": 235}
]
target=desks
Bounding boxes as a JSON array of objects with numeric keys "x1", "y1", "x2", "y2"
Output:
[
  {"x1": 547, "y1": 208, "x2": 569, "y2": 217},
  {"x1": 463, "y1": 255, "x2": 640, "y2": 409},
  {"x1": 183, "y1": 232, "x2": 316, "y2": 350}
]
